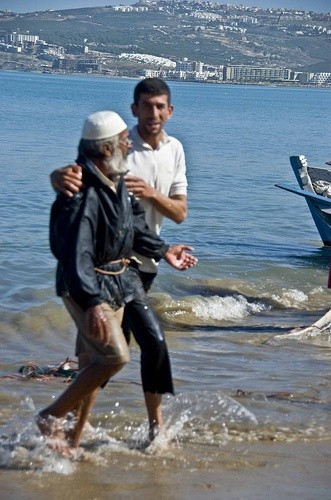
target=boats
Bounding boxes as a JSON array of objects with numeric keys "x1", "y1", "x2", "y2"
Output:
[{"x1": 287, "y1": 155, "x2": 331, "y2": 247}]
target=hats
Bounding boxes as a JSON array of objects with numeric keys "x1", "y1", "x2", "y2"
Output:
[{"x1": 81, "y1": 110, "x2": 127, "y2": 141}]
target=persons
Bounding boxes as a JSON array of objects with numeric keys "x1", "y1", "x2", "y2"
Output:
[
  {"x1": 69, "y1": 77, "x2": 188, "y2": 443},
  {"x1": 30, "y1": 110, "x2": 200, "y2": 461}
]
